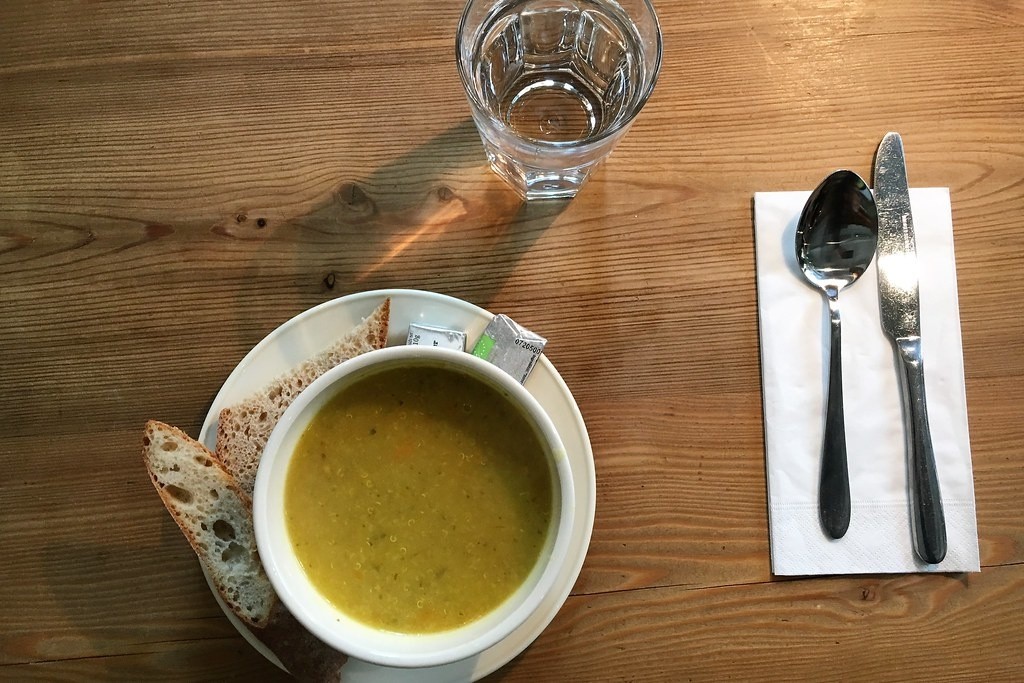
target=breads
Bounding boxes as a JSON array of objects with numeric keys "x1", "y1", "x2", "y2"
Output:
[
  {"x1": 139, "y1": 420, "x2": 351, "y2": 683},
  {"x1": 213, "y1": 298, "x2": 391, "y2": 499}
]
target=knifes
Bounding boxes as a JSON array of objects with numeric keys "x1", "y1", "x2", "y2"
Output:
[{"x1": 875, "y1": 131, "x2": 948, "y2": 565}]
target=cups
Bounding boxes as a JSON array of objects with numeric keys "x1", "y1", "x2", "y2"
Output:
[{"x1": 455, "y1": 0, "x2": 664, "y2": 204}]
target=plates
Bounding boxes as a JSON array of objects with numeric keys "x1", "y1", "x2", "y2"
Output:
[{"x1": 197, "y1": 289, "x2": 597, "y2": 682}]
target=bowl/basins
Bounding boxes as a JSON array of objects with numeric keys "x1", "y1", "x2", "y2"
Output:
[{"x1": 251, "y1": 344, "x2": 574, "y2": 668}]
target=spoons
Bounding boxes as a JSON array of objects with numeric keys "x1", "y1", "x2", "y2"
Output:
[{"x1": 795, "y1": 170, "x2": 880, "y2": 539}]
